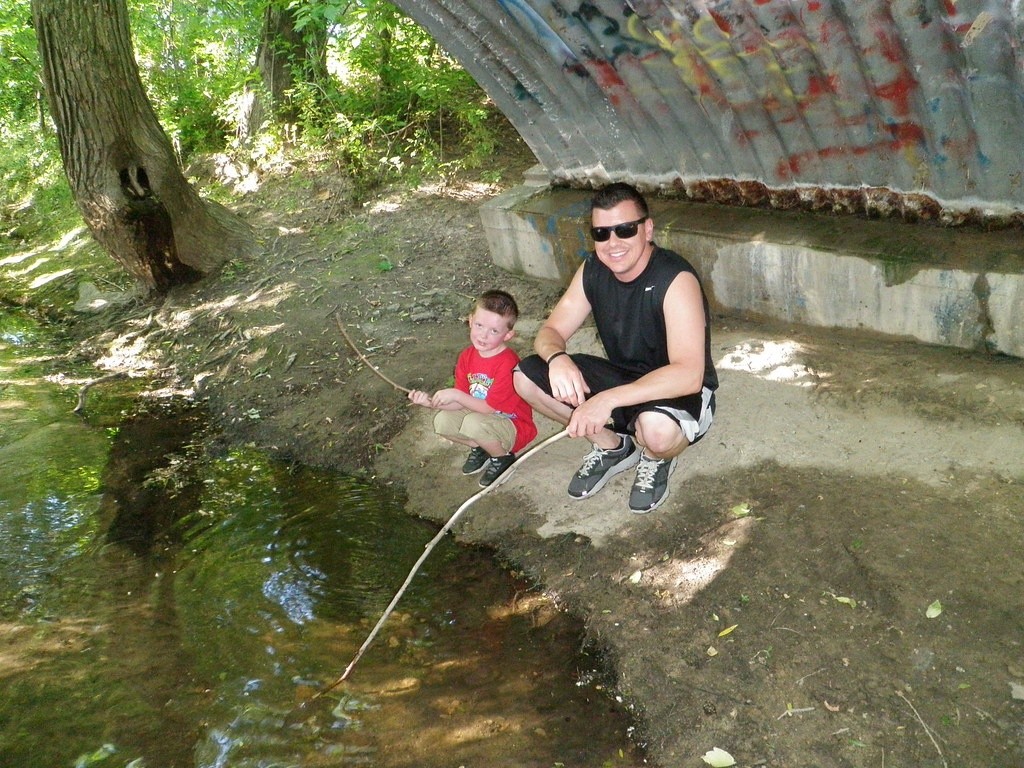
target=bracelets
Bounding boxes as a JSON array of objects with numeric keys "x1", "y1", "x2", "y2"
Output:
[{"x1": 547, "y1": 350, "x2": 567, "y2": 365}]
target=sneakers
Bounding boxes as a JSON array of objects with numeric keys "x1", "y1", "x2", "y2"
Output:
[
  {"x1": 462, "y1": 446, "x2": 492, "y2": 476},
  {"x1": 629, "y1": 446, "x2": 678, "y2": 514},
  {"x1": 478, "y1": 452, "x2": 516, "y2": 488},
  {"x1": 568, "y1": 435, "x2": 641, "y2": 500}
]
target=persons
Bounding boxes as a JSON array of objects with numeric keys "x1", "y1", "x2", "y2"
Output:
[
  {"x1": 511, "y1": 180, "x2": 720, "y2": 515},
  {"x1": 406, "y1": 290, "x2": 538, "y2": 489}
]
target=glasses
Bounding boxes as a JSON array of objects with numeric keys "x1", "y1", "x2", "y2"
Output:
[{"x1": 591, "y1": 215, "x2": 650, "y2": 242}]
